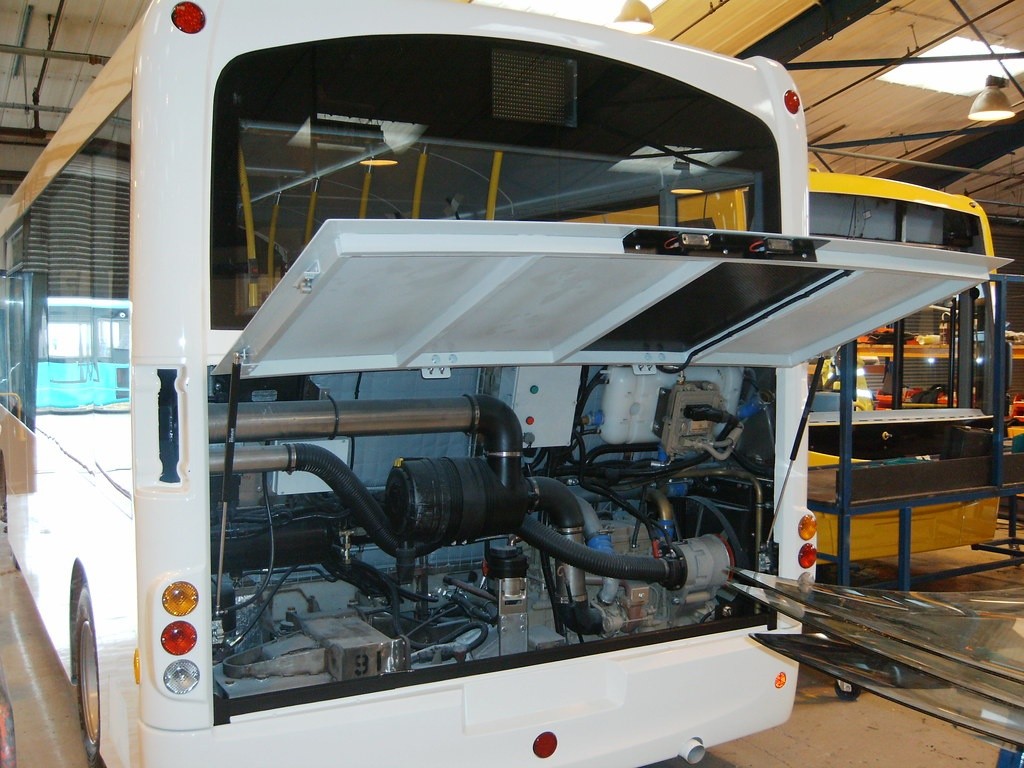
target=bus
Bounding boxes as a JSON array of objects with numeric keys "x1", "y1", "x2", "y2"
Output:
[
  {"x1": 558, "y1": 166, "x2": 1000, "y2": 573},
  {"x1": 0, "y1": 0, "x2": 1015, "y2": 768}
]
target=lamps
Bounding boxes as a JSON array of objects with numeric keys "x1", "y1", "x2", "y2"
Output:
[
  {"x1": 360, "y1": 131, "x2": 398, "y2": 165},
  {"x1": 670, "y1": 162, "x2": 703, "y2": 194},
  {"x1": 968, "y1": 75, "x2": 1016, "y2": 121},
  {"x1": 613, "y1": 0, "x2": 655, "y2": 35}
]
